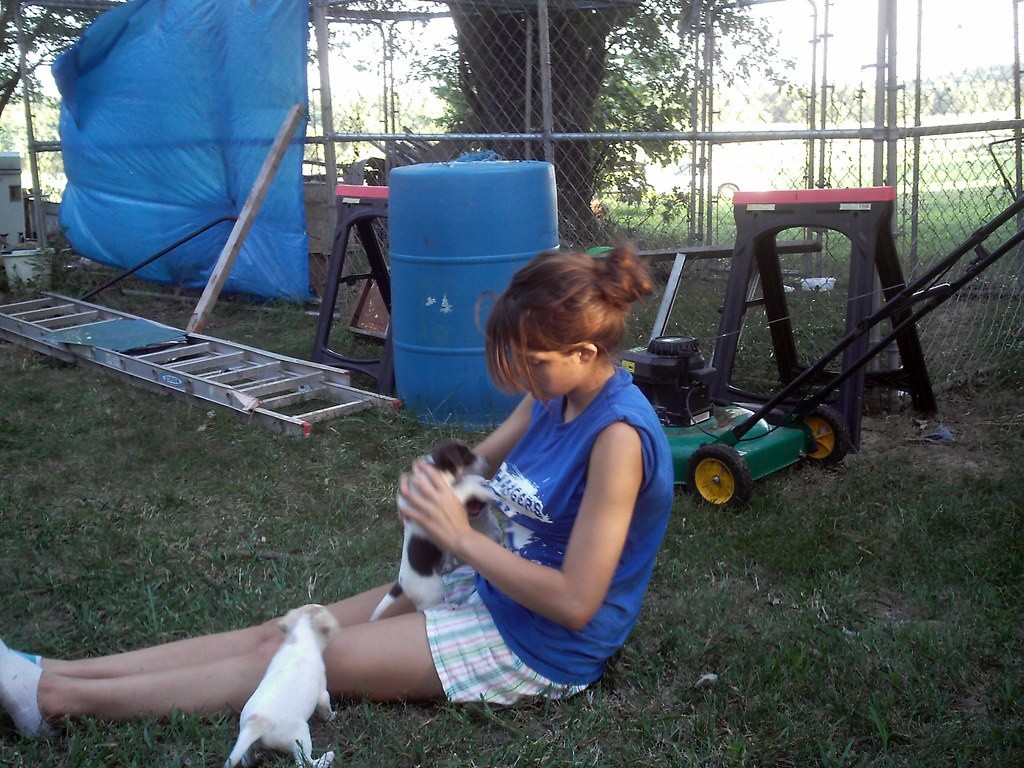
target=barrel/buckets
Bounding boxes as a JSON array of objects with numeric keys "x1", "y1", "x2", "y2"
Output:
[
  {"x1": 388, "y1": 162, "x2": 561, "y2": 431},
  {"x1": 3, "y1": 248, "x2": 54, "y2": 294}
]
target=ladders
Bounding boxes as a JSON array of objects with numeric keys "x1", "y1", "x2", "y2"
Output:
[
  {"x1": 344, "y1": 239, "x2": 824, "y2": 421},
  {"x1": 0, "y1": 283, "x2": 403, "y2": 438}
]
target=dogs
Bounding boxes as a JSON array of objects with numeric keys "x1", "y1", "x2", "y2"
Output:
[
  {"x1": 222, "y1": 603, "x2": 340, "y2": 768},
  {"x1": 369, "y1": 437, "x2": 499, "y2": 623}
]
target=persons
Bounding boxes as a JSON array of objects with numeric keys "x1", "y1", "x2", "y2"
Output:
[{"x1": 0, "y1": 240, "x2": 674, "y2": 741}]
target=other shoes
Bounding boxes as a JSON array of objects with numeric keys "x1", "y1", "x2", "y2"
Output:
[{"x1": 0, "y1": 638, "x2": 61, "y2": 741}]
